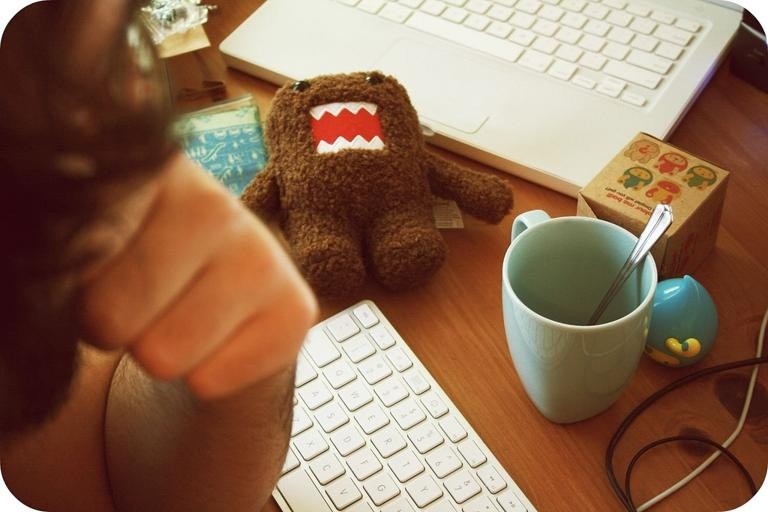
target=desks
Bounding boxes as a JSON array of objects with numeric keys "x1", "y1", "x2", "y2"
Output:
[{"x1": 154, "y1": 1, "x2": 764, "y2": 510}]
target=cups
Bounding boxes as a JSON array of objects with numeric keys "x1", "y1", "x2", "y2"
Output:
[{"x1": 502, "y1": 209, "x2": 657, "y2": 425}]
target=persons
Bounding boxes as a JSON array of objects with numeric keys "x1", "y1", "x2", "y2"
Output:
[{"x1": 0, "y1": 1, "x2": 322, "y2": 511}]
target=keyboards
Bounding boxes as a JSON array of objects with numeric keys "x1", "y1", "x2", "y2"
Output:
[{"x1": 271, "y1": 299, "x2": 540, "y2": 512}]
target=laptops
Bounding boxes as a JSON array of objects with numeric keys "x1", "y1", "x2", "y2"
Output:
[{"x1": 217, "y1": 0, "x2": 745, "y2": 207}]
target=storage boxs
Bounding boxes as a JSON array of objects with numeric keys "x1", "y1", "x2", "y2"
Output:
[{"x1": 576, "y1": 133, "x2": 730, "y2": 279}]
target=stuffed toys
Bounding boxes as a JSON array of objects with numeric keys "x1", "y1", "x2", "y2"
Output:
[{"x1": 239, "y1": 71, "x2": 514, "y2": 302}]
